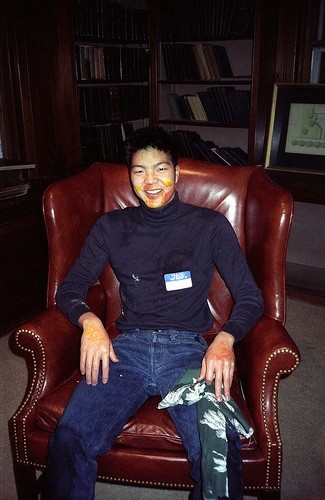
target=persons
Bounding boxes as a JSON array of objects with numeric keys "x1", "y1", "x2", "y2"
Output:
[{"x1": 41, "y1": 126, "x2": 264, "y2": 500}]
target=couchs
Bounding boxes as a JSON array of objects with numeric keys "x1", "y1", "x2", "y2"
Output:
[{"x1": 7, "y1": 158, "x2": 300, "y2": 500}]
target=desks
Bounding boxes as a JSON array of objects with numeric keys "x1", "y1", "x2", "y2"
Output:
[{"x1": 0, "y1": 177, "x2": 72, "y2": 339}]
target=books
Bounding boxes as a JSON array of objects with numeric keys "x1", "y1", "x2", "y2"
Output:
[
  {"x1": 77, "y1": 126, "x2": 248, "y2": 168},
  {"x1": 0, "y1": 158, "x2": 35, "y2": 201},
  {"x1": 75, "y1": 0, "x2": 254, "y2": 43},
  {"x1": 69, "y1": 43, "x2": 253, "y2": 84},
  {"x1": 74, "y1": 86, "x2": 251, "y2": 125}
]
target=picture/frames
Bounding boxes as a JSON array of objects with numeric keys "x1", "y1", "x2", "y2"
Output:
[{"x1": 264, "y1": 83, "x2": 325, "y2": 174}]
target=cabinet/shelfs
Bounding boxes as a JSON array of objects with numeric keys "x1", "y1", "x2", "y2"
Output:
[{"x1": 0, "y1": 0, "x2": 325, "y2": 180}]
